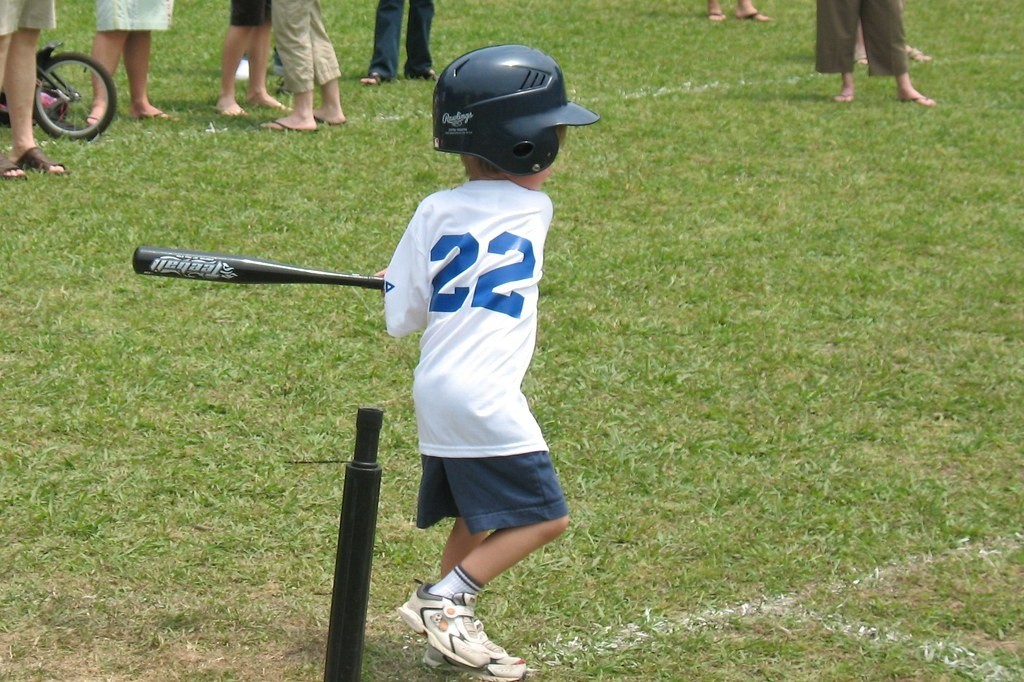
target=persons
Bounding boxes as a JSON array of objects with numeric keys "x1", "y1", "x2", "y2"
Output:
[
  {"x1": 260, "y1": 0, "x2": 346, "y2": 132},
  {"x1": 376, "y1": 44, "x2": 600, "y2": 682},
  {"x1": 0, "y1": 0, "x2": 71, "y2": 179},
  {"x1": 707, "y1": 0, "x2": 768, "y2": 22},
  {"x1": 360, "y1": 0, "x2": 440, "y2": 84},
  {"x1": 814, "y1": 0, "x2": 937, "y2": 107},
  {"x1": 86, "y1": 0, "x2": 175, "y2": 126},
  {"x1": 216, "y1": 0, "x2": 293, "y2": 116}
]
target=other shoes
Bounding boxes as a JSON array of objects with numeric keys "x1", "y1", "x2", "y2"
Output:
[
  {"x1": 40, "y1": 92, "x2": 58, "y2": 106},
  {"x1": 276, "y1": 75, "x2": 292, "y2": 96}
]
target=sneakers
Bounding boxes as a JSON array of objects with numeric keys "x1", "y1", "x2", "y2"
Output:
[
  {"x1": 397, "y1": 578, "x2": 490, "y2": 669},
  {"x1": 423, "y1": 620, "x2": 526, "y2": 682}
]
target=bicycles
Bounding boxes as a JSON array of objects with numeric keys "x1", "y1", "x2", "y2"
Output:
[{"x1": 33, "y1": 41, "x2": 117, "y2": 143}]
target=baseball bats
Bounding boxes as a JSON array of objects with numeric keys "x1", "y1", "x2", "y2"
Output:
[{"x1": 131, "y1": 243, "x2": 384, "y2": 290}]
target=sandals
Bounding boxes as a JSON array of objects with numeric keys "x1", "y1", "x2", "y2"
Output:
[
  {"x1": 905, "y1": 46, "x2": 931, "y2": 62},
  {"x1": 360, "y1": 72, "x2": 385, "y2": 86},
  {"x1": 854, "y1": 47, "x2": 869, "y2": 66},
  {"x1": 405, "y1": 70, "x2": 438, "y2": 82}
]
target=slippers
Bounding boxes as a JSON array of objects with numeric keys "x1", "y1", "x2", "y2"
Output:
[
  {"x1": 735, "y1": 12, "x2": 771, "y2": 22},
  {"x1": 260, "y1": 119, "x2": 319, "y2": 134},
  {"x1": 86, "y1": 103, "x2": 108, "y2": 126},
  {"x1": 896, "y1": 91, "x2": 936, "y2": 108},
  {"x1": 708, "y1": 6, "x2": 726, "y2": 21},
  {"x1": 15, "y1": 147, "x2": 70, "y2": 175},
  {"x1": 314, "y1": 112, "x2": 346, "y2": 126},
  {"x1": 134, "y1": 110, "x2": 175, "y2": 120},
  {"x1": 833, "y1": 84, "x2": 855, "y2": 102},
  {"x1": 0, "y1": 158, "x2": 28, "y2": 181}
]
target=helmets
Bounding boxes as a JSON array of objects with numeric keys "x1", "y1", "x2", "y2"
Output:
[{"x1": 432, "y1": 43, "x2": 600, "y2": 176}]
